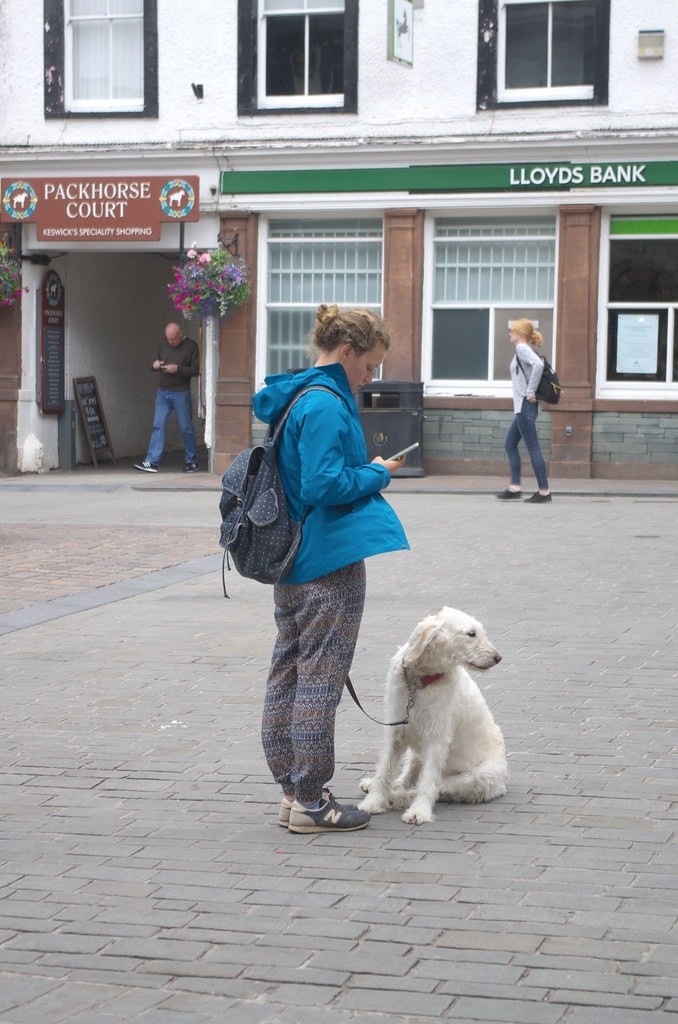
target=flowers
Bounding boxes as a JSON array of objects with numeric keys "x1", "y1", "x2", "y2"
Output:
[
  {"x1": 0, "y1": 231, "x2": 30, "y2": 307},
  {"x1": 167, "y1": 241, "x2": 252, "y2": 322}
]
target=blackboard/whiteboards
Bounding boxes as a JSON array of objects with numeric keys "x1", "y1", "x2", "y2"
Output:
[
  {"x1": 73, "y1": 376, "x2": 112, "y2": 455},
  {"x1": 354, "y1": 381, "x2": 426, "y2": 478}
]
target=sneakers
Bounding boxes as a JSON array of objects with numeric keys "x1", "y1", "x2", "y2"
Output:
[
  {"x1": 132, "y1": 461, "x2": 158, "y2": 472},
  {"x1": 524, "y1": 491, "x2": 552, "y2": 503},
  {"x1": 288, "y1": 788, "x2": 371, "y2": 832},
  {"x1": 496, "y1": 489, "x2": 522, "y2": 501},
  {"x1": 183, "y1": 463, "x2": 199, "y2": 472},
  {"x1": 277, "y1": 797, "x2": 358, "y2": 827}
]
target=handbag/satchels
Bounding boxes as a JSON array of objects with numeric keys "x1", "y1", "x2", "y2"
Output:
[{"x1": 515, "y1": 349, "x2": 563, "y2": 404}]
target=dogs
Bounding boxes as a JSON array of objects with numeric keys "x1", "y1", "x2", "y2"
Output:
[{"x1": 356, "y1": 605, "x2": 508, "y2": 826}]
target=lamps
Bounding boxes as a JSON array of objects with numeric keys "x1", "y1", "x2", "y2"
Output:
[{"x1": 638, "y1": 29, "x2": 665, "y2": 60}]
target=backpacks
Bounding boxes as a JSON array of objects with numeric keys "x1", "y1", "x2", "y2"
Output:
[{"x1": 219, "y1": 385, "x2": 342, "y2": 599}]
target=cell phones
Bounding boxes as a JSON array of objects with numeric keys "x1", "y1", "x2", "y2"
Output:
[{"x1": 389, "y1": 443, "x2": 419, "y2": 461}]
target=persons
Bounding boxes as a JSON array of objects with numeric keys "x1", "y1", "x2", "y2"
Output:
[
  {"x1": 133, "y1": 324, "x2": 200, "y2": 475},
  {"x1": 496, "y1": 319, "x2": 553, "y2": 503},
  {"x1": 250, "y1": 303, "x2": 410, "y2": 833}
]
